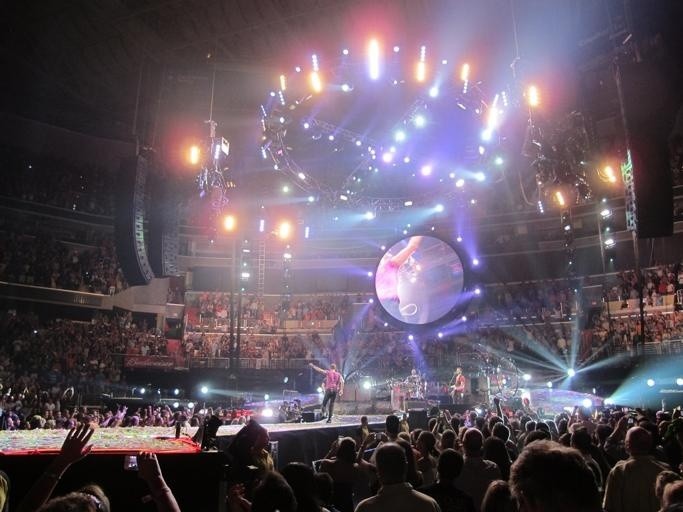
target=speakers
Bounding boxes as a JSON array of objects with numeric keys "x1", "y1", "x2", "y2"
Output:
[
  {"x1": 405, "y1": 392, "x2": 472, "y2": 429},
  {"x1": 608, "y1": 63, "x2": 683, "y2": 240},
  {"x1": 302, "y1": 412, "x2": 321, "y2": 422},
  {"x1": 115, "y1": 154, "x2": 180, "y2": 287}
]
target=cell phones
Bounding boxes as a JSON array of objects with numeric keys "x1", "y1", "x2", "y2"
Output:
[
  {"x1": 338, "y1": 435, "x2": 344, "y2": 446},
  {"x1": 627, "y1": 417, "x2": 634, "y2": 425},
  {"x1": 374, "y1": 433, "x2": 382, "y2": 440},
  {"x1": 123, "y1": 455, "x2": 139, "y2": 471}
]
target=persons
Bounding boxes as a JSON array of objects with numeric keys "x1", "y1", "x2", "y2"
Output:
[
  {"x1": 386, "y1": 236, "x2": 452, "y2": 323},
  {"x1": 1, "y1": 261, "x2": 683, "y2": 512},
  {"x1": 2, "y1": 151, "x2": 130, "y2": 296}
]
